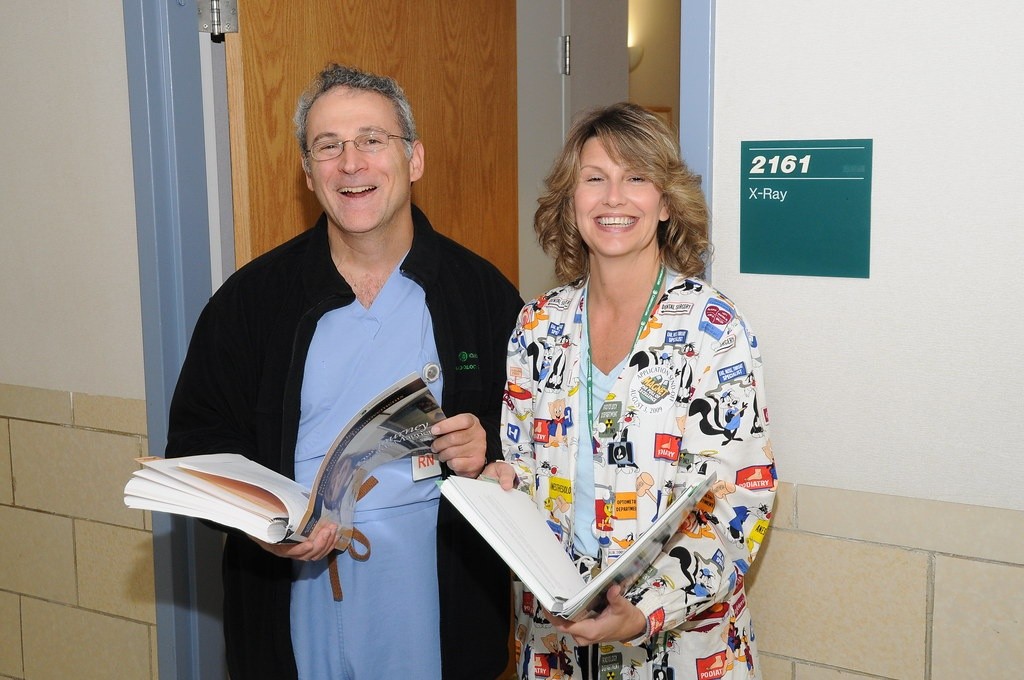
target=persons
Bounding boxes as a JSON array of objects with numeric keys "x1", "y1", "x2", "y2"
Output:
[
  {"x1": 477, "y1": 103, "x2": 777, "y2": 680},
  {"x1": 166, "y1": 64, "x2": 528, "y2": 680}
]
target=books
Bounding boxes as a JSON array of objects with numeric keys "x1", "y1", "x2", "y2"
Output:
[
  {"x1": 439, "y1": 471, "x2": 718, "y2": 623},
  {"x1": 124, "y1": 371, "x2": 448, "y2": 545}
]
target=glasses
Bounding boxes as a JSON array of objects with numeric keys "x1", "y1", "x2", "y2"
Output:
[{"x1": 304, "y1": 131, "x2": 413, "y2": 161}]
